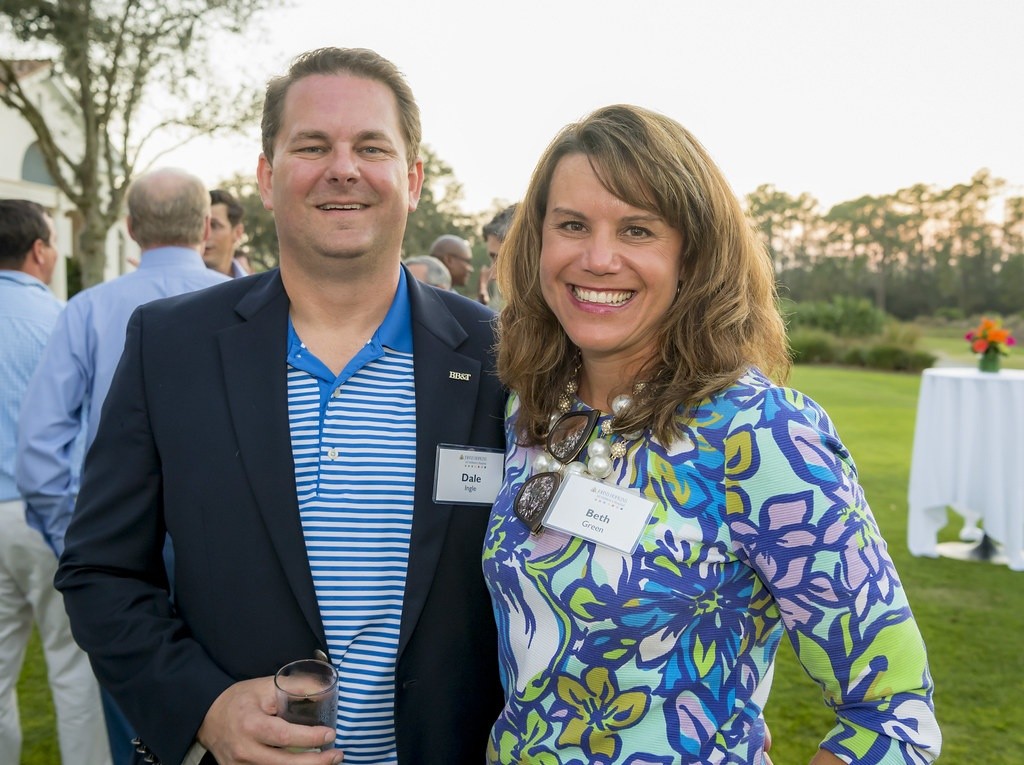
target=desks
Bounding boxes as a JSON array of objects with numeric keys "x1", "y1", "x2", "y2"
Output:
[{"x1": 907, "y1": 367, "x2": 1024, "y2": 572}]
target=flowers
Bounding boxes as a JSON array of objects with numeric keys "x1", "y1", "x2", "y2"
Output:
[{"x1": 965, "y1": 316, "x2": 1016, "y2": 357}]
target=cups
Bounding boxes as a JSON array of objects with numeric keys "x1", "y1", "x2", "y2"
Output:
[{"x1": 273, "y1": 660, "x2": 338, "y2": 764}]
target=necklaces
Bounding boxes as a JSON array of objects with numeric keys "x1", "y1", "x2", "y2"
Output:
[{"x1": 535, "y1": 353, "x2": 676, "y2": 484}]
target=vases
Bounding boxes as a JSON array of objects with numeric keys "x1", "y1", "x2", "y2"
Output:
[{"x1": 979, "y1": 350, "x2": 1000, "y2": 372}]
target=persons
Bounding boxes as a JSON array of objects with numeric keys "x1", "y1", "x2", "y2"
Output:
[
  {"x1": 387, "y1": 192, "x2": 528, "y2": 314},
  {"x1": 53, "y1": 47, "x2": 509, "y2": 764},
  {"x1": 479, "y1": 104, "x2": 942, "y2": 765},
  {"x1": 0, "y1": 169, "x2": 248, "y2": 765}
]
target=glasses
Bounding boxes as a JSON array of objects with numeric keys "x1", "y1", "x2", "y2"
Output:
[
  {"x1": 448, "y1": 253, "x2": 473, "y2": 264},
  {"x1": 513, "y1": 409, "x2": 601, "y2": 538}
]
